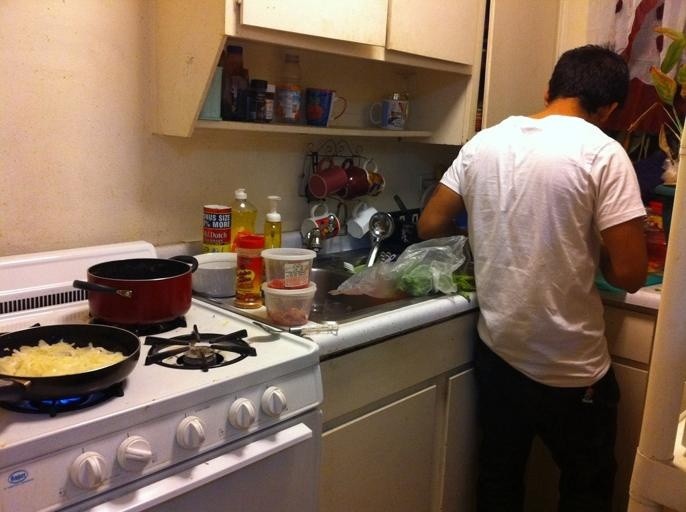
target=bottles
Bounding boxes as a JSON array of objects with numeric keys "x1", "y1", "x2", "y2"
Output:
[
  {"x1": 220, "y1": 44, "x2": 249, "y2": 119},
  {"x1": 228, "y1": 188, "x2": 257, "y2": 247},
  {"x1": 277, "y1": 50, "x2": 304, "y2": 122}
]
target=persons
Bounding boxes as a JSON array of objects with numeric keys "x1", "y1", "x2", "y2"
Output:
[{"x1": 416, "y1": 41, "x2": 650, "y2": 512}]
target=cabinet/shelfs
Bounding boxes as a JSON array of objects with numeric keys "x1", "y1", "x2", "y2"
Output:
[
  {"x1": 442, "y1": 367, "x2": 538, "y2": 512},
  {"x1": 473, "y1": 0, "x2": 560, "y2": 145},
  {"x1": 594, "y1": 304, "x2": 660, "y2": 512},
  {"x1": 146, "y1": 0, "x2": 481, "y2": 148},
  {"x1": 318, "y1": 384, "x2": 441, "y2": 512}
]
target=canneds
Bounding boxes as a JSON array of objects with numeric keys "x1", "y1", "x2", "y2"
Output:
[
  {"x1": 265, "y1": 84, "x2": 276, "y2": 122},
  {"x1": 233, "y1": 235, "x2": 265, "y2": 308}
]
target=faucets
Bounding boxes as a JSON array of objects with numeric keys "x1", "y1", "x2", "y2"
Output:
[{"x1": 303, "y1": 227, "x2": 322, "y2": 252}]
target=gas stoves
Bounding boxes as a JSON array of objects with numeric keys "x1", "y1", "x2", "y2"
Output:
[{"x1": 0, "y1": 239, "x2": 324, "y2": 512}]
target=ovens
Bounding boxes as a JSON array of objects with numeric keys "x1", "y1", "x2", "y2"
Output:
[{"x1": 0, "y1": 409, "x2": 325, "y2": 512}]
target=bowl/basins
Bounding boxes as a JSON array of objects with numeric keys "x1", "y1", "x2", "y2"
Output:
[{"x1": 187, "y1": 250, "x2": 240, "y2": 297}]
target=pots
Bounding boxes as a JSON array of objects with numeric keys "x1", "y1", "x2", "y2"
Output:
[
  {"x1": 0, "y1": 323, "x2": 141, "y2": 403},
  {"x1": 73, "y1": 252, "x2": 198, "y2": 324}
]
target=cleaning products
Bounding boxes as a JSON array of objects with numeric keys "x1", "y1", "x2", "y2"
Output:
[
  {"x1": 265, "y1": 194, "x2": 283, "y2": 248},
  {"x1": 230, "y1": 188, "x2": 257, "y2": 239}
]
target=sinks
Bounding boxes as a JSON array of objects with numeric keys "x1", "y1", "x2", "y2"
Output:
[{"x1": 308, "y1": 267, "x2": 443, "y2": 327}]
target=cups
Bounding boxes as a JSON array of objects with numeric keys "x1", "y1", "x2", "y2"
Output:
[
  {"x1": 298, "y1": 156, "x2": 386, "y2": 240},
  {"x1": 305, "y1": 85, "x2": 345, "y2": 126},
  {"x1": 369, "y1": 98, "x2": 410, "y2": 130}
]
